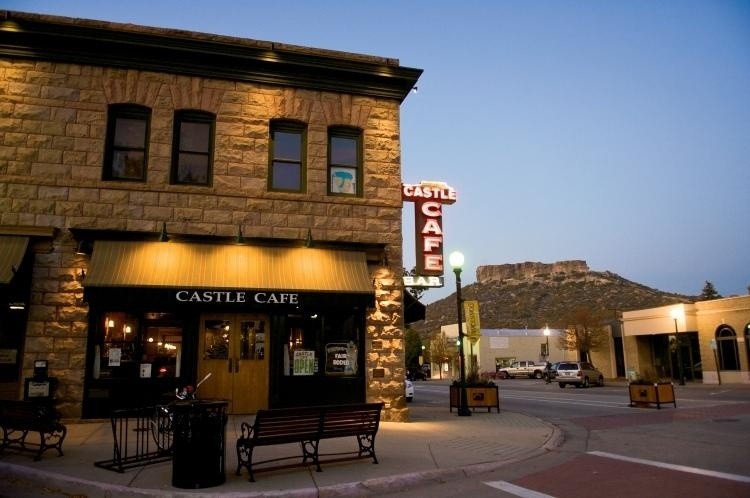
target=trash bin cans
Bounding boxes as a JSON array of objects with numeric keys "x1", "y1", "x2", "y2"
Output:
[{"x1": 167, "y1": 399, "x2": 230, "y2": 489}]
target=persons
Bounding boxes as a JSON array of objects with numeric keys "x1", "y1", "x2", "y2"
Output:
[{"x1": 544, "y1": 360, "x2": 552, "y2": 382}]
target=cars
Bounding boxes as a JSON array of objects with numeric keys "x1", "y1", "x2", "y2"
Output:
[{"x1": 406, "y1": 379, "x2": 414, "y2": 401}]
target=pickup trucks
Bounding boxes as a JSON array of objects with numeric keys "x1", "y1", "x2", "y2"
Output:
[{"x1": 499, "y1": 360, "x2": 548, "y2": 380}]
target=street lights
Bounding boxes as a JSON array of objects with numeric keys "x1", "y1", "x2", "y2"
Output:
[
  {"x1": 543, "y1": 322, "x2": 550, "y2": 356},
  {"x1": 670, "y1": 309, "x2": 685, "y2": 385},
  {"x1": 449, "y1": 251, "x2": 472, "y2": 417}
]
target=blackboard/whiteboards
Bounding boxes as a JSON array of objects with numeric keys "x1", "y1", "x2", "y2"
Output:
[{"x1": 325, "y1": 343, "x2": 358, "y2": 376}]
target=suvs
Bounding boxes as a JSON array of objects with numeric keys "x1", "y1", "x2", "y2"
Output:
[
  {"x1": 555, "y1": 360, "x2": 603, "y2": 387},
  {"x1": 406, "y1": 367, "x2": 428, "y2": 381}
]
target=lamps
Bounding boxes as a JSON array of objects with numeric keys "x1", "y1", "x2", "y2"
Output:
[
  {"x1": 157, "y1": 222, "x2": 173, "y2": 243},
  {"x1": 235, "y1": 226, "x2": 246, "y2": 246},
  {"x1": 74, "y1": 239, "x2": 89, "y2": 256},
  {"x1": 304, "y1": 229, "x2": 314, "y2": 247},
  {"x1": 33, "y1": 359, "x2": 48, "y2": 376}
]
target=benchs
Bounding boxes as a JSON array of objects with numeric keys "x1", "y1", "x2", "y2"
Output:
[
  {"x1": 233, "y1": 401, "x2": 385, "y2": 482},
  {"x1": 0, "y1": 399, "x2": 67, "y2": 462}
]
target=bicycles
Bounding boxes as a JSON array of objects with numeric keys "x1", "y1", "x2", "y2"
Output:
[{"x1": 151, "y1": 384, "x2": 198, "y2": 450}]
